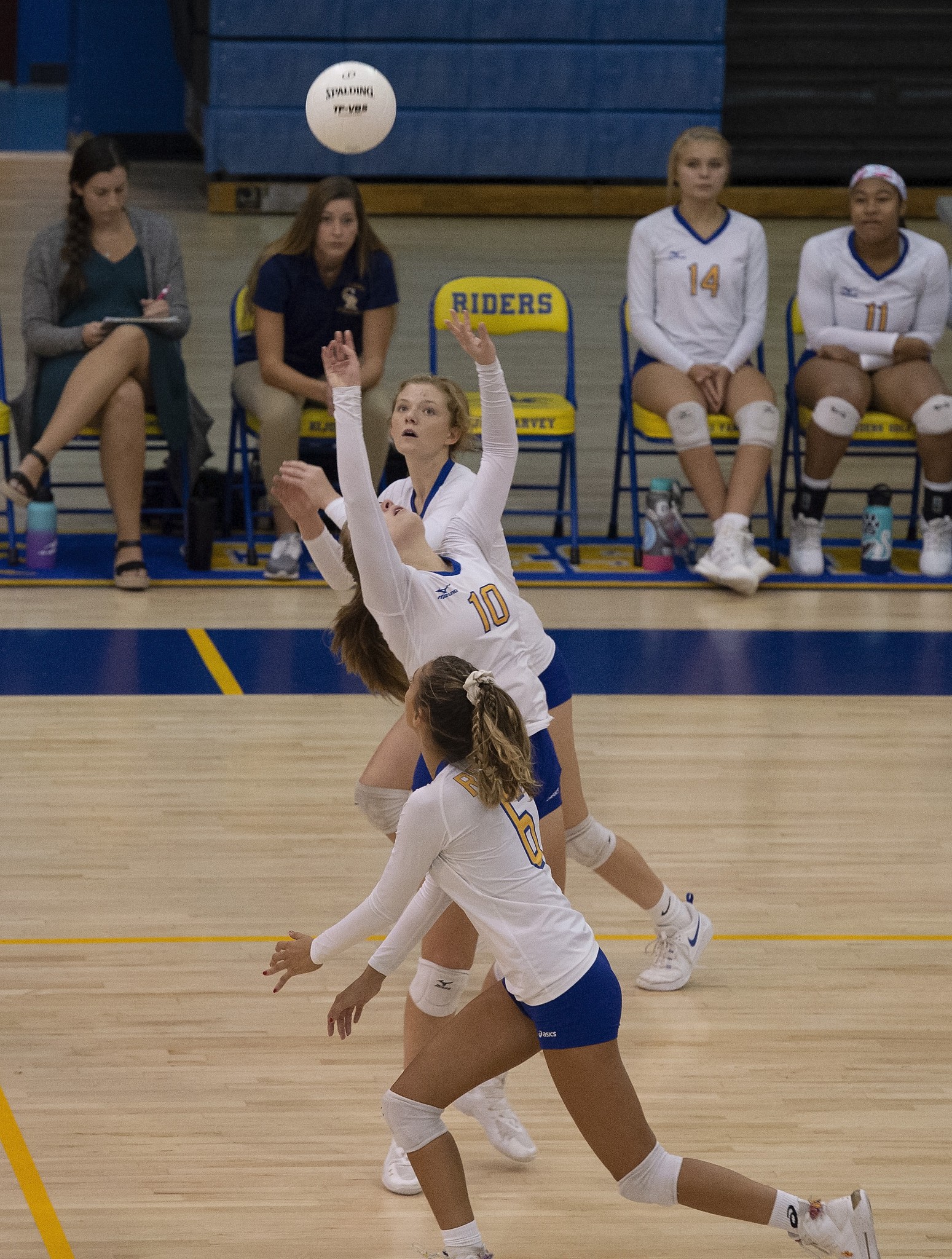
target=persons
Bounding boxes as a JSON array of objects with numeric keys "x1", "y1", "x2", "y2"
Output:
[
  {"x1": 628, "y1": 122, "x2": 781, "y2": 594},
  {"x1": 227, "y1": 165, "x2": 402, "y2": 580},
  {"x1": 0, "y1": 135, "x2": 208, "y2": 592},
  {"x1": 309, "y1": 309, "x2": 568, "y2": 1199},
  {"x1": 261, "y1": 373, "x2": 712, "y2": 995},
  {"x1": 781, "y1": 160, "x2": 951, "y2": 579},
  {"x1": 255, "y1": 655, "x2": 884, "y2": 1259}
]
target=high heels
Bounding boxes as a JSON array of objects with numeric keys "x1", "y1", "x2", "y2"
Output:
[
  {"x1": 112, "y1": 539, "x2": 149, "y2": 589},
  {"x1": 0, "y1": 447, "x2": 50, "y2": 508}
]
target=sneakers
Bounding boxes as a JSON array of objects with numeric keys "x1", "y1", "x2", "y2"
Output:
[
  {"x1": 788, "y1": 512, "x2": 827, "y2": 576},
  {"x1": 452, "y1": 1070, "x2": 539, "y2": 1162},
  {"x1": 694, "y1": 524, "x2": 775, "y2": 594},
  {"x1": 635, "y1": 892, "x2": 713, "y2": 991},
  {"x1": 787, "y1": 1189, "x2": 881, "y2": 1259},
  {"x1": 263, "y1": 531, "x2": 303, "y2": 579},
  {"x1": 917, "y1": 507, "x2": 952, "y2": 577},
  {"x1": 381, "y1": 1140, "x2": 422, "y2": 1196},
  {"x1": 411, "y1": 1240, "x2": 494, "y2": 1259}
]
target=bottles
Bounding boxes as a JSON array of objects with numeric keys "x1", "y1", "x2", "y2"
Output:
[
  {"x1": 860, "y1": 484, "x2": 893, "y2": 573},
  {"x1": 26, "y1": 487, "x2": 57, "y2": 570},
  {"x1": 643, "y1": 478, "x2": 675, "y2": 571}
]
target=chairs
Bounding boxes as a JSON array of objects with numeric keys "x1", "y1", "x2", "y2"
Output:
[{"x1": 0, "y1": 275, "x2": 919, "y2": 567}]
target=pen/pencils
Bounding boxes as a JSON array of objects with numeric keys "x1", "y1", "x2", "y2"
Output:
[{"x1": 156, "y1": 283, "x2": 172, "y2": 300}]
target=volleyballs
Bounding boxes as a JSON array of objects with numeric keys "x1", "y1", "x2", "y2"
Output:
[{"x1": 305, "y1": 61, "x2": 397, "y2": 155}]
